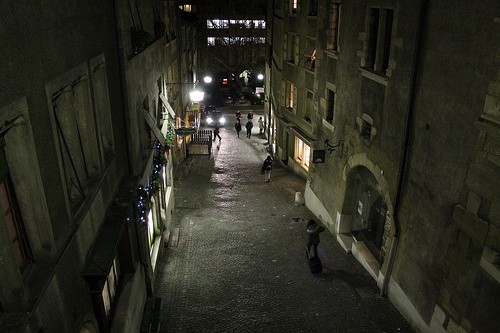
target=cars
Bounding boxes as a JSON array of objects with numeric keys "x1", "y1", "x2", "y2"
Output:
[{"x1": 203, "y1": 106, "x2": 225, "y2": 126}]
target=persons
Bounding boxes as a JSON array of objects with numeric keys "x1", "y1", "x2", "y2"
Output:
[
  {"x1": 258, "y1": 117, "x2": 265, "y2": 134},
  {"x1": 246, "y1": 111, "x2": 254, "y2": 122},
  {"x1": 263, "y1": 156, "x2": 272, "y2": 183},
  {"x1": 235, "y1": 109, "x2": 241, "y2": 122},
  {"x1": 213, "y1": 124, "x2": 222, "y2": 141},
  {"x1": 246, "y1": 120, "x2": 253, "y2": 139},
  {"x1": 304, "y1": 219, "x2": 325, "y2": 259},
  {"x1": 235, "y1": 121, "x2": 242, "y2": 138}
]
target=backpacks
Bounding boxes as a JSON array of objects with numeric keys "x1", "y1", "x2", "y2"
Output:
[{"x1": 267, "y1": 164, "x2": 272, "y2": 169}]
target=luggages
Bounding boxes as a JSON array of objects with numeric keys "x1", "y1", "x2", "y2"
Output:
[{"x1": 305, "y1": 246, "x2": 323, "y2": 274}]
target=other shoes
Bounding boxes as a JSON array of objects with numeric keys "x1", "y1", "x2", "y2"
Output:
[{"x1": 268, "y1": 179, "x2": 269, "y2": 182}]
